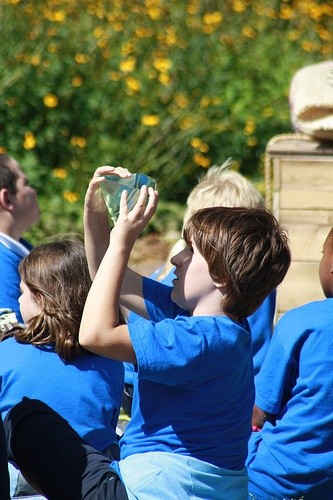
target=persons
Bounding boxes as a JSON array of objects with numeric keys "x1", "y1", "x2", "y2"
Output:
[
  {"x1": 245, "y1": 227, "x2": 333, "y2": 500},
  {"x1": 0, "y1": 232, "x2": 126, "y2": 500},
  {"x1": 3, "y1": 165, "x2": 291, "y2": 500},
  {"x1": 121, "y1": 157, "x2": 277, "y2": 419},
  {"x1": 0, "y1": 153, "x2": 41, "y2": 325}
]
